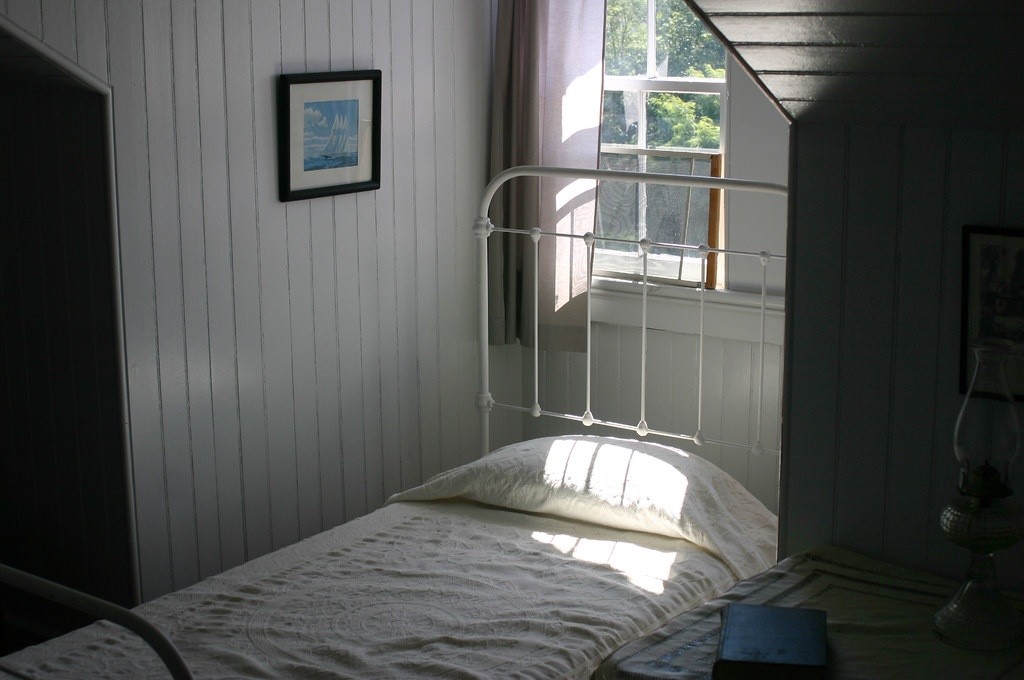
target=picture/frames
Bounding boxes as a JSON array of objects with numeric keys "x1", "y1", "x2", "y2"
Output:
[
  {"x1": 958, "y1": 223, "x2": 1024, "y2": 403},
  {"x1": 275, "y1": 69, "x2": 383, "y2": 203}
]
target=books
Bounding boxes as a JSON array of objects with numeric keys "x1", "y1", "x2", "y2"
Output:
[{"x1": 712, "y1": 603, "x2": 828, "y2": 680}]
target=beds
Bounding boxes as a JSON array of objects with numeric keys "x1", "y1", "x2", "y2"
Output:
[{"x1": 0, "y1": 162, "x2": 791, "y2": 680}]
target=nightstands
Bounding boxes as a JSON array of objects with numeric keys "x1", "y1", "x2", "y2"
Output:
[{"x1": 595, "y1": 544, "x2": 1024, "y2": 680}]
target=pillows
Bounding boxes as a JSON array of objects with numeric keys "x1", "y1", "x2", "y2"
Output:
[{"x1": 389, "y1": 431, "x2": 778, "y2": 581}]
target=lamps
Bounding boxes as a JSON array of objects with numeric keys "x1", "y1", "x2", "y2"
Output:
[{"x1": 929, "y1": 337, "x2": 1024, "y2": 653}]
